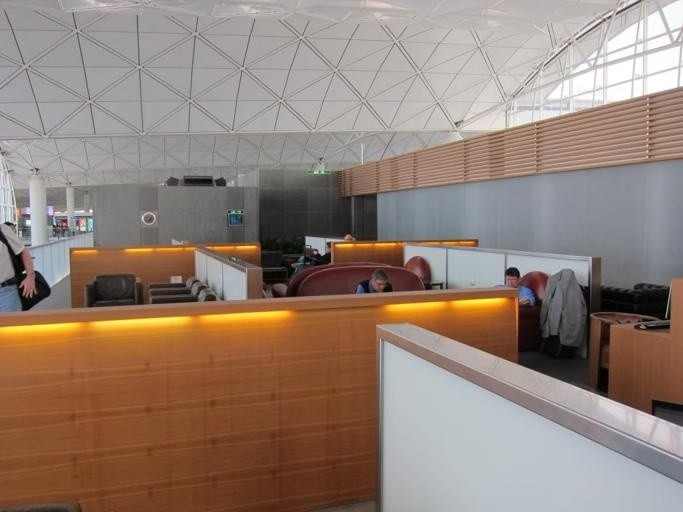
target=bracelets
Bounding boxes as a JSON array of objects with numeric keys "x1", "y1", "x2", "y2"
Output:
[{"x1": 27, "y1": 271, "x2": 36, "y2": 277}]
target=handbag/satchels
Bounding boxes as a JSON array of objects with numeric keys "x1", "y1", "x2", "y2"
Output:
[{"x1": 18, "y1": 271, "x2": 50, "y2": 310}]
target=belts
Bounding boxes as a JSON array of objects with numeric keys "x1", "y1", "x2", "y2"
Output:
[{"x1": 0, "y1": 278, "x2": 17, "y2": 288}]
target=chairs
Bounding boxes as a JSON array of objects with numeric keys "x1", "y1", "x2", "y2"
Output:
[
  {"x1": 600, "y1": 283, "x2": 669, "y2": 318},
  {"x1": 85, "y1": 275, "x2": 218, "y2": 305},
  {"x1": 518, "y1": 271, "x2": 549, "y2": 351}
]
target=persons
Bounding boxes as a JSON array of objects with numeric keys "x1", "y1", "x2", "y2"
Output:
[
  {"x1": 297, "y1": 234, "x2": 356, "y2": 265},
  {"x1": 4, "y1": 222, "x2": 16, "y2": 234},
  {"x1": 356, "y1": 269, "x2": 394, "y2": 294},
  {"x1": 495, "y1": 268, "x2": 536, "y2": 306},
  {"x1": 0, "y1": 223, "x2": 38, "y2": 313}
]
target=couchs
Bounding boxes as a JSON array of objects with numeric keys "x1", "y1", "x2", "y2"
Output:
[{"x1": 271, "y1": 262, "x2": 427, "y2": 296}]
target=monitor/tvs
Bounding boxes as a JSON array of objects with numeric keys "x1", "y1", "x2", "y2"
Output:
[{"x1": 228, "y1": 213, "x2": 244, "y2": 226}]
